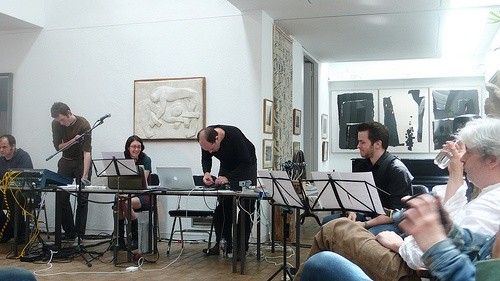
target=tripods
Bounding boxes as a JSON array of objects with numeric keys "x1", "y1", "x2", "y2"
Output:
[
  {"x1": 256, "y1": 171, "x2": 305, "y2": 281},
  {"x1": 42, "y1": 121, "x2": 157, "y2": 268}
]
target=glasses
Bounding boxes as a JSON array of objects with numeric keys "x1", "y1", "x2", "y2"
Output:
[{"x1": 129, "y1": 144, "x2": 141, "y2": 149}]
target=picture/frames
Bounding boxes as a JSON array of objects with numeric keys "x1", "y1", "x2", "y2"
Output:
[
  {"x1": 322, "y1": 141, "x2": 329, "y2": 161},
  {"x1": 133, "y1": 77, "x2": 206, "y2": 139},
  {"x1": 293, "y1": 109, "x2": 302, "y2": 135},
  {"x1": 263, "y1": 99, "x2": 275, "y2": 134},
  {"x1": 321, "y1": 114, "x2": 329, "y2": 139},
  {"x1": 263, "y1": 139, "x2": 274, "y2": 167}
]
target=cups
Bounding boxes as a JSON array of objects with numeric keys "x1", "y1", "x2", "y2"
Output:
[{"x1": 434, "y1": 135, "x2": 464, "y2": 169}]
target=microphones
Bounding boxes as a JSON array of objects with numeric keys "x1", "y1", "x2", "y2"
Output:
[{"x1": 97, "y1": 113, "x2": 111, "y2": 121}]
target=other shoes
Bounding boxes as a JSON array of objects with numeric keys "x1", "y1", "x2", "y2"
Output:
[
  {"x1": 125, "y1": 240, "x2": 138, "y2": 250},
  {"x1": 236, "y1": 245, "x2": 246, "y2": 261},
  {"x1": 69, "y1": 233, "x2": 84, "y2": 241},
  {"x1": 0, "y1": 233, "x2": 14, "y2": 243},
  {"x1": 110, "y1": 240, "x2": 124, "y2": 250},
  {"x1": 61, "y1": 234, "x2": 74, "y2": 240},
  {"x1": 18, "y1": 237, "x2": 26, "y2": 244},
  {"x1": 203, "y1": 238, "x2": 232, "y2": 255}
]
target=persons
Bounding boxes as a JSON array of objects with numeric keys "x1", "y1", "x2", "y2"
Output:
[
  {"x1": 197, "y1": 125, "x2": 257, "y2": 260},
  {"x1": 111, "y1": 136, "x2": 156, "y2": 250},
  {"x1": 303, "y1": 116, "x2": 500, "y2": 281},
  {"x1": 321, "y1": 122, "x2": 414, "y2": 236},
  {"x1": 0, "y1": 135, "x2": 41, "y2": 245},
  {"x1": 292, "y1": 193, "x2": 500, "y2": 281},
  {"x1": 51, "y1": 102, "x2": 92, "y2": 242}
]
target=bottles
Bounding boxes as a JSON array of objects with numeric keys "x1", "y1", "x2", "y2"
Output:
[{"x1": 219, "y1": 237, "x2": 227, "y2": 257}]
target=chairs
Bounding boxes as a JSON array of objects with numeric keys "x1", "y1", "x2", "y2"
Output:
[
  {"x1": 124, "y1": 174, "x2": 161, "y2": 255},
  {"x1": 25, "y1": 194, "x2": 50, "y2": 242},
  {"x1": 166, "y1": 174, "x2": 218, "y2": 254}
]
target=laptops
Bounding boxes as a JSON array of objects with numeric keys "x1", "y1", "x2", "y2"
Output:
[
  {"x1": 156, "y1": 166, "x2": 204, "y2": 190},
  {"x1": 110, "y1": 167, "x2": 158, "y2": 189}
]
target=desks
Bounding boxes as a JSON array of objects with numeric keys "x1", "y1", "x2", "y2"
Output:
[{"x1": 0, "y1": 186, "x2": 271, "y2": 275}]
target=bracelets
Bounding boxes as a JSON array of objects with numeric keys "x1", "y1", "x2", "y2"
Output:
[{"x1": 364, "y1": 220, "x2": 368, "y2": 229}]
